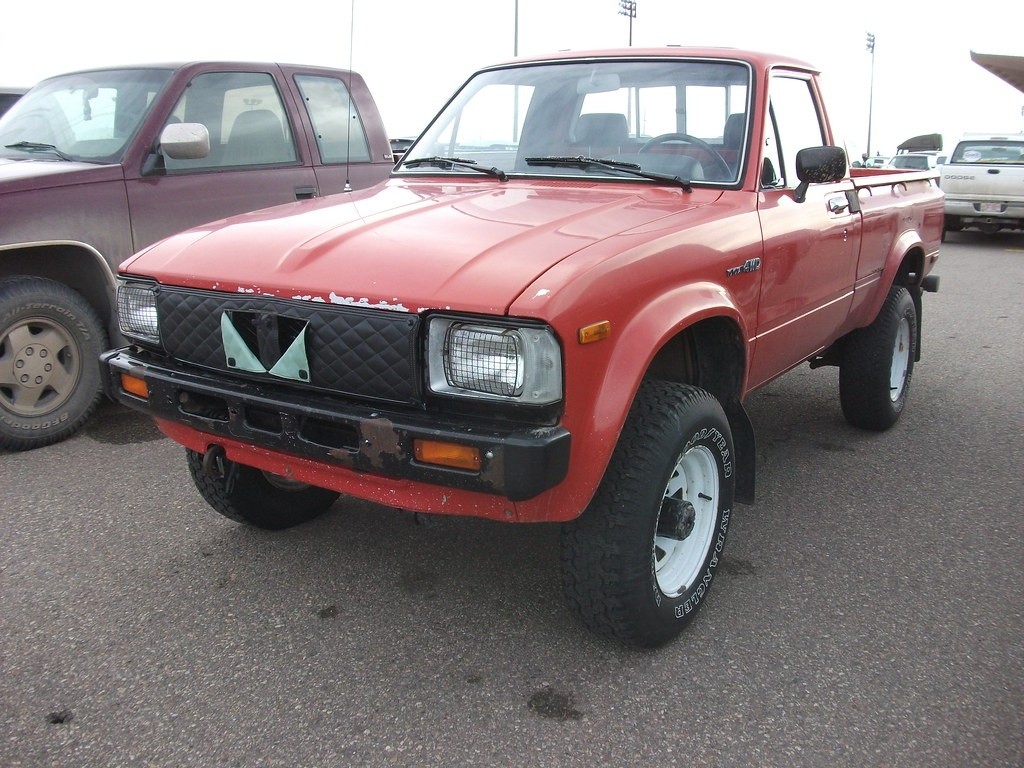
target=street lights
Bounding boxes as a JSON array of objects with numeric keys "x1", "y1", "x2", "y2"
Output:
[
  {"x1": 619, "y1": 0, "x2": 637, "y2": 132},
  {"x1": 865, "y1": 31, "x2": 875, "y2": 156}
]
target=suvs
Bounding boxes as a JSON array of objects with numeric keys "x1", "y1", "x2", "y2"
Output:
[{"x1": 885, "y1": 151, "x2": 948, "y2": 184}]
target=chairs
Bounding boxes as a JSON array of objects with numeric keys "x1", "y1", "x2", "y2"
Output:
[
  {"x1": 575, "y1": 114, "x2": 631, "y2": 170},
  {"x1": 725, "y1": 112, "x2": 748, "y2": 151},
  {"x1": 220, "y1": 109, "x2": 289, "y2": 165}
]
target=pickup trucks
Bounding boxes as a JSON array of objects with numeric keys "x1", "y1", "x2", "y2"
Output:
[
  {"x1": 939, "y1": 135, "x2": 1024, "y2": 242},
  {"x1": 98, "y1": 44, "x2": 947, "y2": 646},
  {"x1": 0, "y1": 62, "x2": 397, "y2": 451}
]
